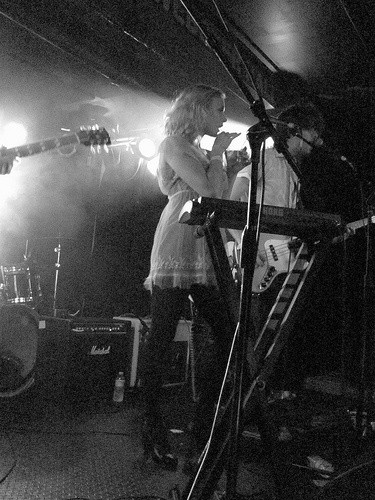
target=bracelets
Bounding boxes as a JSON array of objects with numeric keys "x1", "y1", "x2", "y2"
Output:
[{"x1": 208, "y1": 154, "x2": 223, "y2": 162}]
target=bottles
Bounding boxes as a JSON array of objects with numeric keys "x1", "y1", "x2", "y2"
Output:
[
  {"x1": 113, "y1": 372, "x2": 125, "y2": 403},
  {"x1": 306, "y1": 454, "x2": 335, "y2": 478}
]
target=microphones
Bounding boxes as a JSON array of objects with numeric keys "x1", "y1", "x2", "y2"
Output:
[{"x1": 270, "y1": 117, "x2": 295, "y2": 129}]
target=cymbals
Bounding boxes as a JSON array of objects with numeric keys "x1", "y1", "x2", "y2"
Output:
[{"x1": 37, "y1": 236, "x2": 76, "y2": 241}]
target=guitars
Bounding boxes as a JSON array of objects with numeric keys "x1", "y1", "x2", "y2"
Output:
[
  {"x1": 234, "y1": 215, "x2": 375, "y2": 296},
  {"x1": 0, "y1": 123, "x2": 111, "y2": 164}
]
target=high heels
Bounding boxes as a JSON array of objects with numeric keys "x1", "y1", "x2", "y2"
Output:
[{"x1": 143, "y1": 430, "x2": 178, "y2": 467}]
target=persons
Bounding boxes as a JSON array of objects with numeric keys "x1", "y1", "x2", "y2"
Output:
[
  {"x1": 225, "y1": 99, "x2": 355, "y2": 454},
  {"x1": 141, "y1": 82, "x2": 250, "y2": 464},
  {"x1": 0, "y1": 146, "x2": 16, "y2": 175}
]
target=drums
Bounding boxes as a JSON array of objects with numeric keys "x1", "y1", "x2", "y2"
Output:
[
  {"x1": 0, "y1": 262, "x2": 43, "y2": 304},
  {"x1": 0, "y1": 303, "x2": 43, "y2": 397}
]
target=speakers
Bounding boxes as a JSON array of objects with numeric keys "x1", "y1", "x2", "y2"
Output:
[{"x1": 35, "y1": 314, "x2": 192, "y2": 403}]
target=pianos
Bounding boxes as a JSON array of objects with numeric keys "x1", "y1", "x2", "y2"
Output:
[{"x1": 178, "y1": 197, "x2": 341, "y2": 500}]
